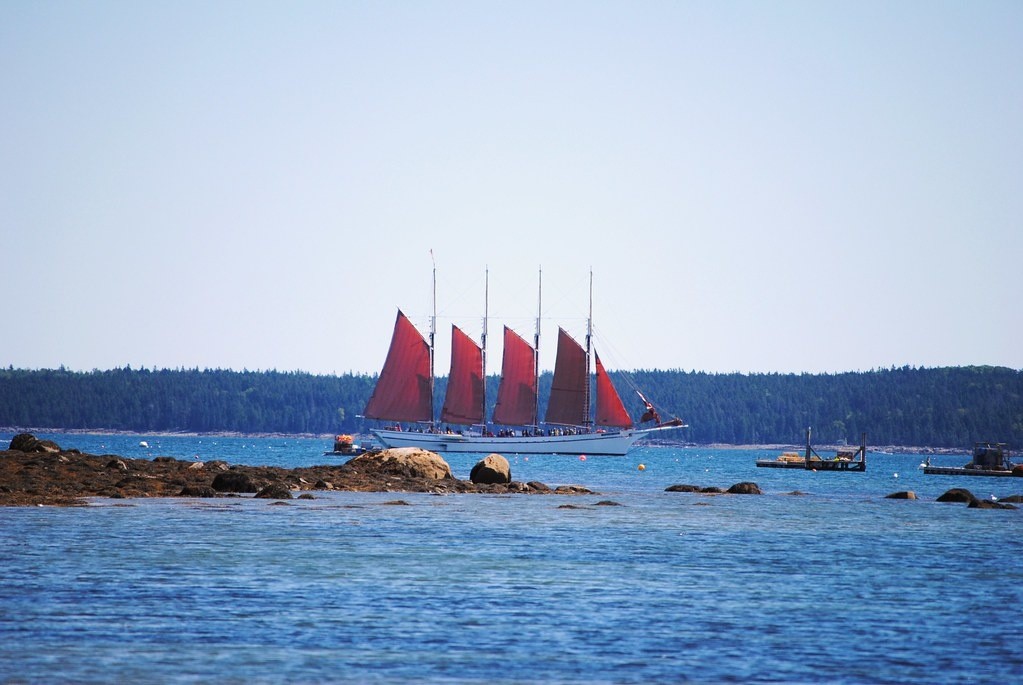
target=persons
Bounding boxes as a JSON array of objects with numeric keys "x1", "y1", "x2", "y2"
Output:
[
  {"x1": 396, "y1": 422, "x2": 401, "y2": 431},
  {"x1": 926, "y1": 456, "x2": 931, "y2": 466},
  {"x1": 496, "y1": 428, "x2": 514, "y2": 437},
  {"x1": 522, "y1": 429, "x2": 544, "y2": 437},
  {"x1": 409, "y1": 425, "x2": 454, "y2": 434},
  {"x1": 548, "y1": 427, "x2": 582, "y2": 436},
  {"x1": 482, "y1": 428, "x2": 488, "y2": 437}
]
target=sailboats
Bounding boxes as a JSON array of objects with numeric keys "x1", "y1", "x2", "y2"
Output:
[{"x1": 355, "y1": 248, "x2": 690, "y2": 456}]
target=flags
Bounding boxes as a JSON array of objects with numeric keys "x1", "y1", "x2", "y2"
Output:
[{"x1": 637, "y1": 391, "x2": 652, "y2": 409}]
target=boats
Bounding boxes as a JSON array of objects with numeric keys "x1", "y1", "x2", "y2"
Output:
[{"x1": 324, "y1": 445, "x2": 383, "y2": 457}]
